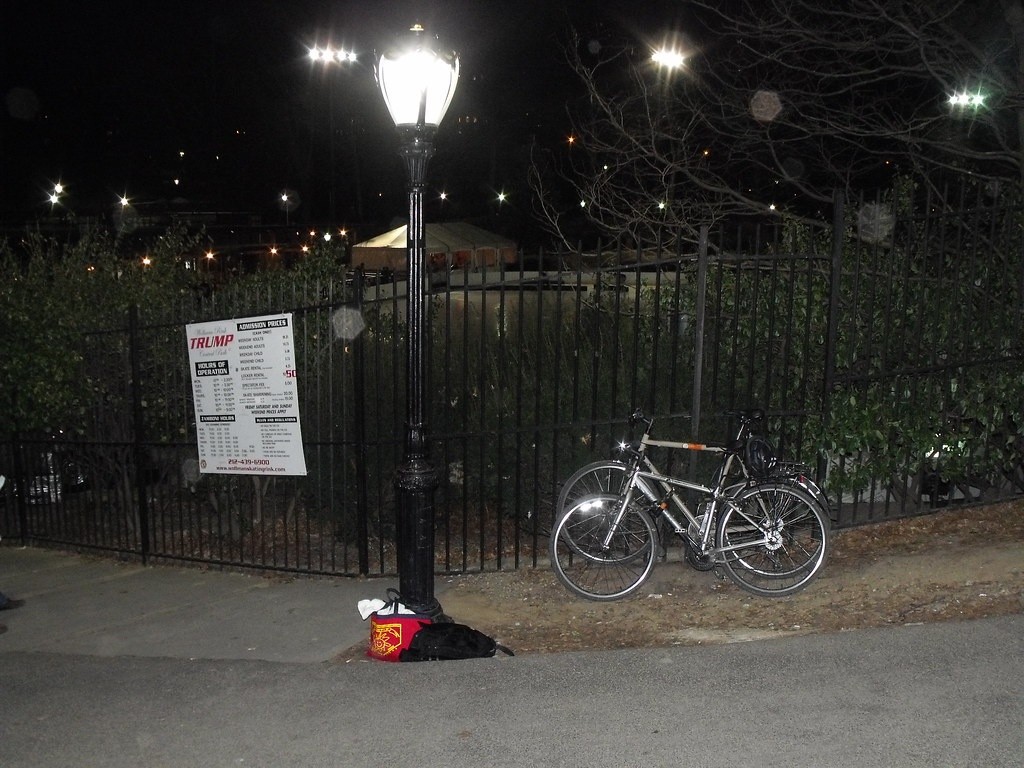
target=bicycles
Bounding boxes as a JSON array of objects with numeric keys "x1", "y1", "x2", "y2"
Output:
[{"x1": 545, "y1": 406, "x2": 832, "y2": 603}]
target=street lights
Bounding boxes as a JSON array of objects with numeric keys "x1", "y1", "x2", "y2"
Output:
[{"x1": 377, "y1": 19, "x2": 462, "y2": 624}]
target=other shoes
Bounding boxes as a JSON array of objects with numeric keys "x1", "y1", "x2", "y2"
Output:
[{"x1": 0, "y1": 591, "x2": 25, "y2": 611}]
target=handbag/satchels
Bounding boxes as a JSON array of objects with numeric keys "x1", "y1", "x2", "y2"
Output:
[
  {"x1": 366, "y1": 589, "x2": 431, "y2": 662},
  {"x1": 397, "y1": 619, "x2": 498, "y2": 662}
]
